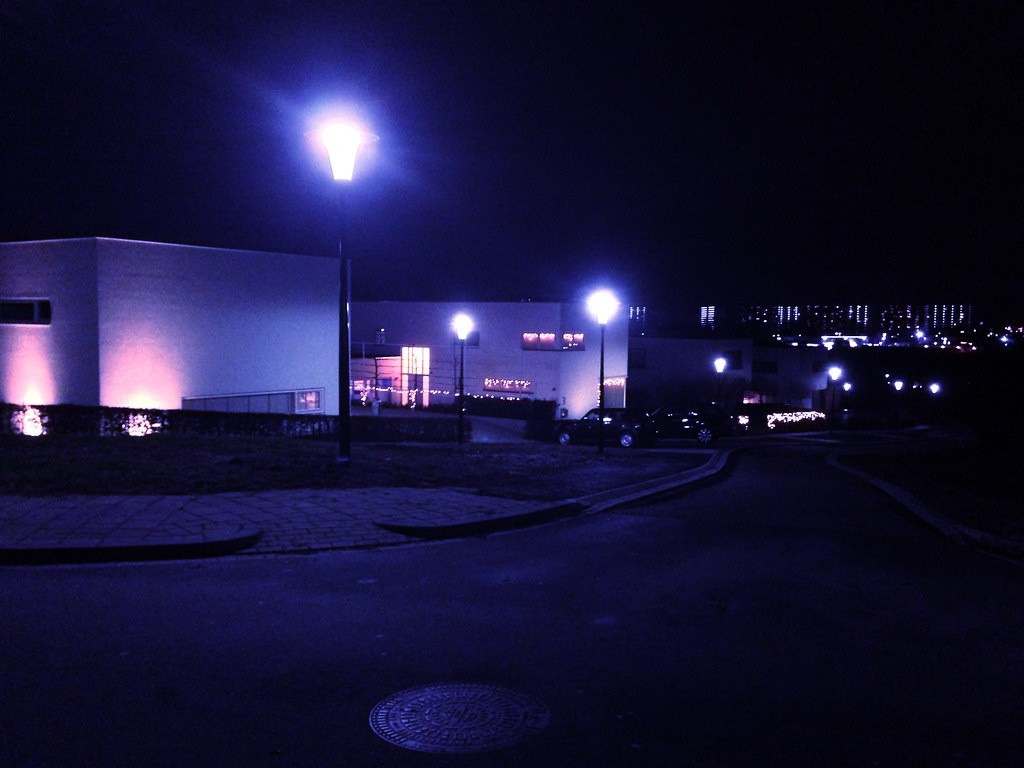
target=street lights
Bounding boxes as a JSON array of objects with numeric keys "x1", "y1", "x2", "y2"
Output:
[
  {"x1": 712, "y1": 356, "x2": 729, "y2": 437},
  {"x1": 447, "y1": 312, "x2": 477, "y2": 444},
  {"x1": 304, "y1": 111, "x2": 382, "y2": 455},
  {"x1": 827, "y1": 366, "x2": 844, "y2": 435},
  {"x1": 845, "y1": 372, "x2": 941, "y2": 433},
  {"x1": 580, "y1": 287, "x2": 623, "y2": 451}
]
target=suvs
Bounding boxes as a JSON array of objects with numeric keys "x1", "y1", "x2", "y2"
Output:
[
  {"x1": 550, "y1": 406, "x2": 662, "y2": 449},
  {"x1": 646, "y1": 400, "x2": 737, "y2": 446}
]
